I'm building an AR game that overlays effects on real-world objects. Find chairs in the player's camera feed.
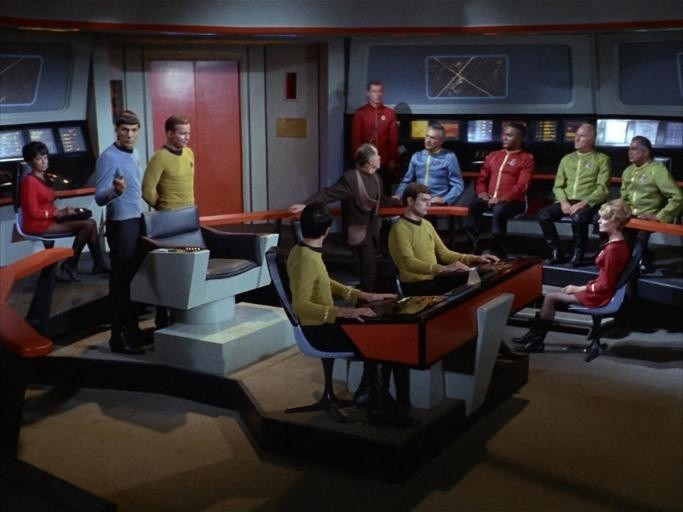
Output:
[264,246,368,426]
[569,243,646,363]
[14,160,67,284]
[373,215,404,287]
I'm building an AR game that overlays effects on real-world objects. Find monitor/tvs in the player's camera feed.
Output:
[410,119,683,148]
[0,129,26,159]
[29,128,58,155]
[58,125,87,153]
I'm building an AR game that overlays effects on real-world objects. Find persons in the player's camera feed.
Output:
[393,122,466,205]
[350,79,398,193]
[143,114,196,325]
[618,135,682,263]
[19,140,105,279]
[93,109,149,355]
[292,143,385,267]
[389,180,501,353]
[512,199,635,353]
[468,115,536,252]
[540,122,614,266]
[286,204,399,417]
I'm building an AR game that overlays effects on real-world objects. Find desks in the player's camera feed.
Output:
[341,256,543,427]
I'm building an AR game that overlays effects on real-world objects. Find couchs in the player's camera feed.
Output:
[112,206,298,378]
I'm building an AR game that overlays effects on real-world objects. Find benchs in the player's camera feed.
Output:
[0,246,79,359]
[599,213,682,237]
[196,205,470,244]
[454,171,683,225]
